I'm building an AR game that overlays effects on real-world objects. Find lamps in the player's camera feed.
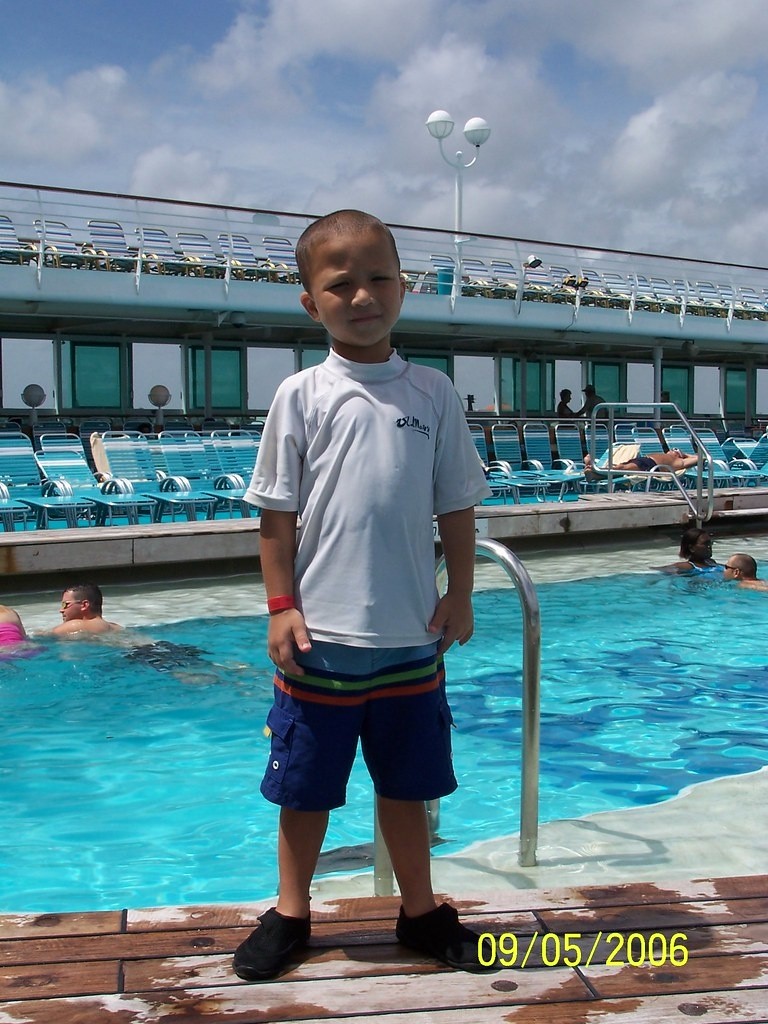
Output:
[517,255,543,315]
[562,274,589,330]
[680,338,699,357]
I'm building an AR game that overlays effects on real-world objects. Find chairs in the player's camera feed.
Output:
[430,254,768,322]
[0,215,303,285]
[0,418,768,533]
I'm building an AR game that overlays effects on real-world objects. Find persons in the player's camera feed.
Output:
[583,448,699,483]
[574,384,608,427]
[0,603,27,645]
[34,583,123,634]
[233,209,491,983]
[556,389,575,428]
[675,529,757,580]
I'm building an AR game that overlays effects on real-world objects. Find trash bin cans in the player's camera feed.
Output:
[437,268,453,296]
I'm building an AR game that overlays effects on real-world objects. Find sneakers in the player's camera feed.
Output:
[231,906,314,979]
[394,901,500,967]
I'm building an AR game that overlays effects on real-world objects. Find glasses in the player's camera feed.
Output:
[671,447,683,458]
[61,599,85,610]
[725,563,737,570]
[697,540,714,548]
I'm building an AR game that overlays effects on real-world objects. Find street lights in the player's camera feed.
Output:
[426,110,490,296]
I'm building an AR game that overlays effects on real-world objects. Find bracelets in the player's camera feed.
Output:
[266,596,295,612]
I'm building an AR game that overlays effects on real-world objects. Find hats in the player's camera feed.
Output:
[582,385,595,391]
